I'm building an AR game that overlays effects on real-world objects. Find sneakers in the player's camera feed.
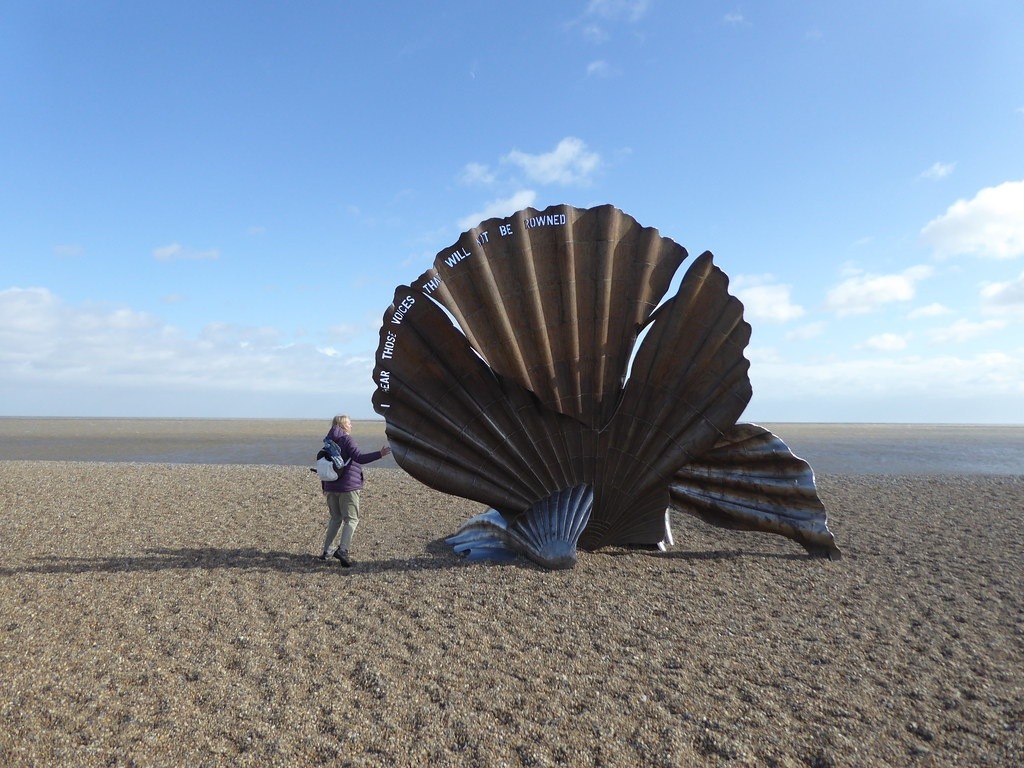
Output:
[318,552,330,563]
[333,545,353,567]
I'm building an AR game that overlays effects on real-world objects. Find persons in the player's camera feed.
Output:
[320,416,392,567]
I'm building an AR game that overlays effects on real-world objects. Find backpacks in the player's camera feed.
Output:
[310,438,352,481]
